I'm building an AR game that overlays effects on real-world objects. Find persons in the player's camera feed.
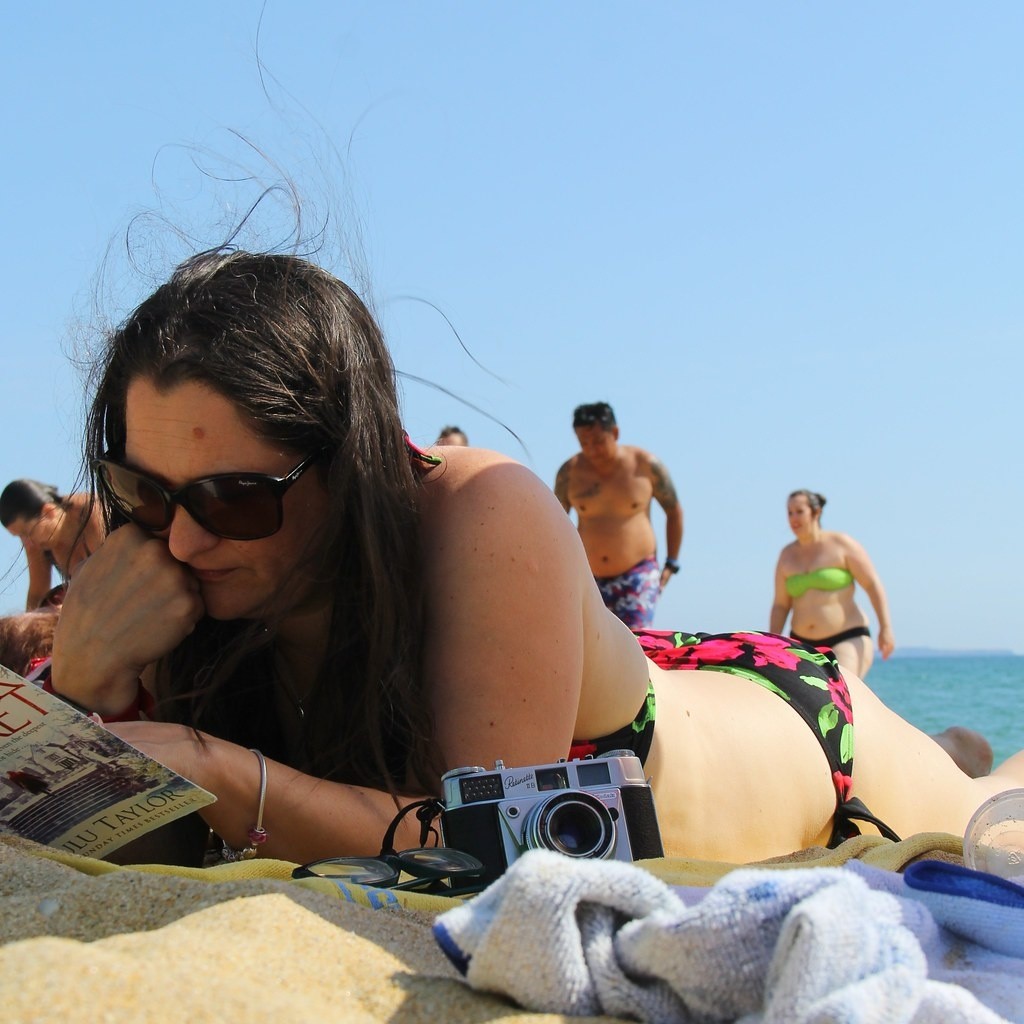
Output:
[47,244,1024,863]
[768,490,894,680]
[554,401,684,631]
[437,426,468,446]
[0,479,112,612]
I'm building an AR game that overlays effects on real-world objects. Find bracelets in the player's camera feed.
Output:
[216,747,268,862]
[40,673,154,721]
[665,558,680,573]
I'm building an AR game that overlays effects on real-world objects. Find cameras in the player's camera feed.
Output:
[437,749,665,899]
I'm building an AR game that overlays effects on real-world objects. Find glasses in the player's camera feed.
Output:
[90,448,326,542]
[291,847,499,897]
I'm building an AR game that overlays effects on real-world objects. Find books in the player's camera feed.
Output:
[0,663,218,861]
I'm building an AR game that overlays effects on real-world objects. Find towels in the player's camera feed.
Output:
[432,847,1024,1024]
